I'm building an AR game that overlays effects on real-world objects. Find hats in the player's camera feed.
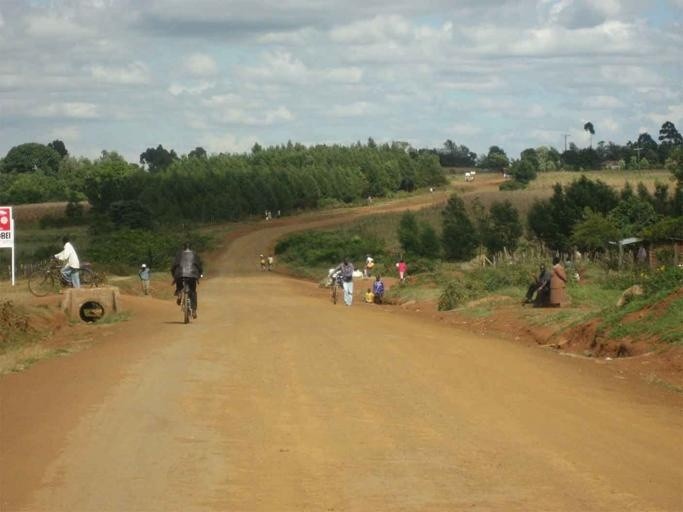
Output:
[142,264,146,268]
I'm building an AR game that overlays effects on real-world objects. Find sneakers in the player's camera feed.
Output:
[176,298,180,305]
[192,311,196,319]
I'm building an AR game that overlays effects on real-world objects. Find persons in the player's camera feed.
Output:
[50,236,81,289]
[170,241,203,319]
[260,253,274,271]
[524,257,581,308]
[362,253,407,305]
[636,243,647,265]
[138,263,152,295]
[329,257,354,305]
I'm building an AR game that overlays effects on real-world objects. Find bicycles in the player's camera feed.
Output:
[178,283,194,325]
[329,276,341,303]
[28,257,95,297]
